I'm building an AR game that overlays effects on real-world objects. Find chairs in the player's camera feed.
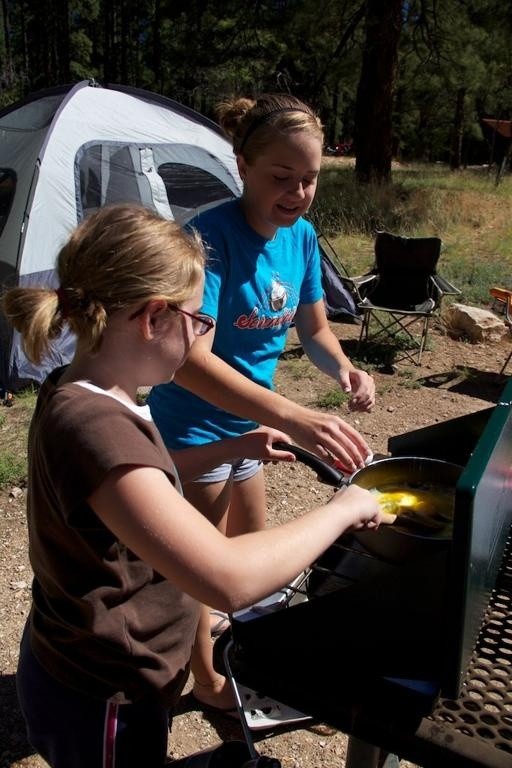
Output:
[353,229,461,366]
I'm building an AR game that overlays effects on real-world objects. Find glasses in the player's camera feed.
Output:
[130,294,213,337]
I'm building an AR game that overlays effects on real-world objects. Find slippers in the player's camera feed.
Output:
[206,609,231,632]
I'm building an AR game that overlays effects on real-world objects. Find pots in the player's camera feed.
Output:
[272,440,469,569]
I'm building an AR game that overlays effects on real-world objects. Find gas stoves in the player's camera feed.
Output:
[211,376,512,701]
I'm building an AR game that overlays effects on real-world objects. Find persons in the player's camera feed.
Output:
[145,95,377,723]
[0,199,384,768]
